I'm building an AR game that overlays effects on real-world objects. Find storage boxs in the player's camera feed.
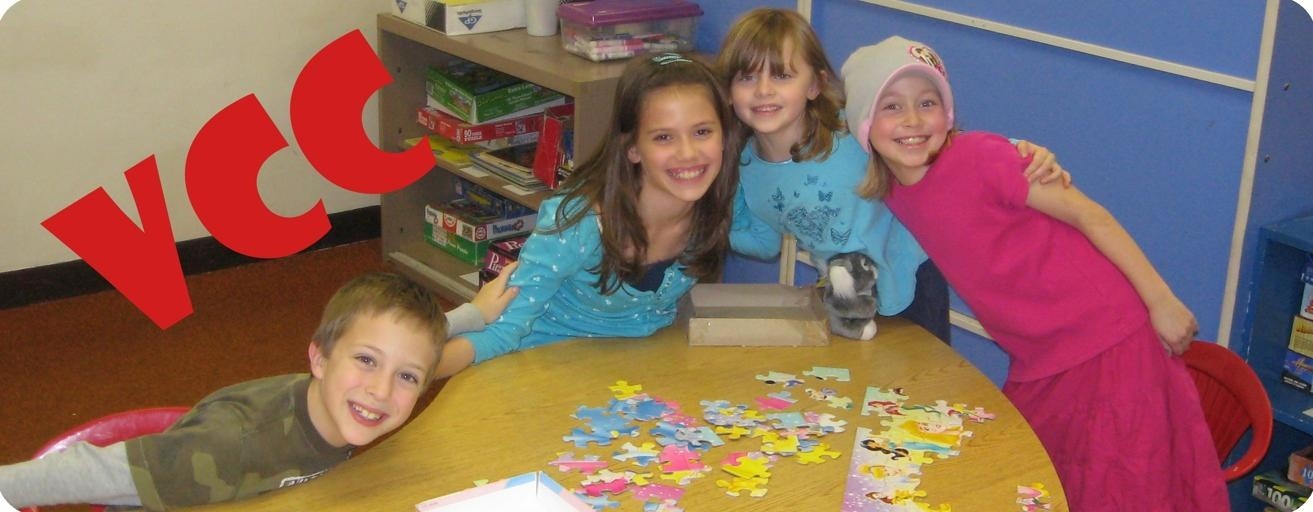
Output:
[560,1,704,61]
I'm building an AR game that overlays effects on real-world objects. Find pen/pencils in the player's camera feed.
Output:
[559,33,694,63]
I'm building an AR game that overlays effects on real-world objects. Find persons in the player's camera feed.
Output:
[839,32,1233,511]
[1,260,520,508]
[431,49,744,383]
[714,6,1072,341]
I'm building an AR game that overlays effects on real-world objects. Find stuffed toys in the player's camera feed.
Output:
[545,366,1056,511]
[821,251,877,341]
[546,365,1055,511]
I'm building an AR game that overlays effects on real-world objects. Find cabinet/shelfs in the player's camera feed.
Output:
[373,9,722,314]
[1249,212,1313,512]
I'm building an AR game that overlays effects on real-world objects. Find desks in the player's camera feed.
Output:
[210,306,1072,512]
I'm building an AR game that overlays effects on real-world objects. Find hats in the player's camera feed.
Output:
[841,36,955,154]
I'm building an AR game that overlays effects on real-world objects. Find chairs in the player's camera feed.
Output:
[1171,336,1278,486]
[2,405,204,509]
